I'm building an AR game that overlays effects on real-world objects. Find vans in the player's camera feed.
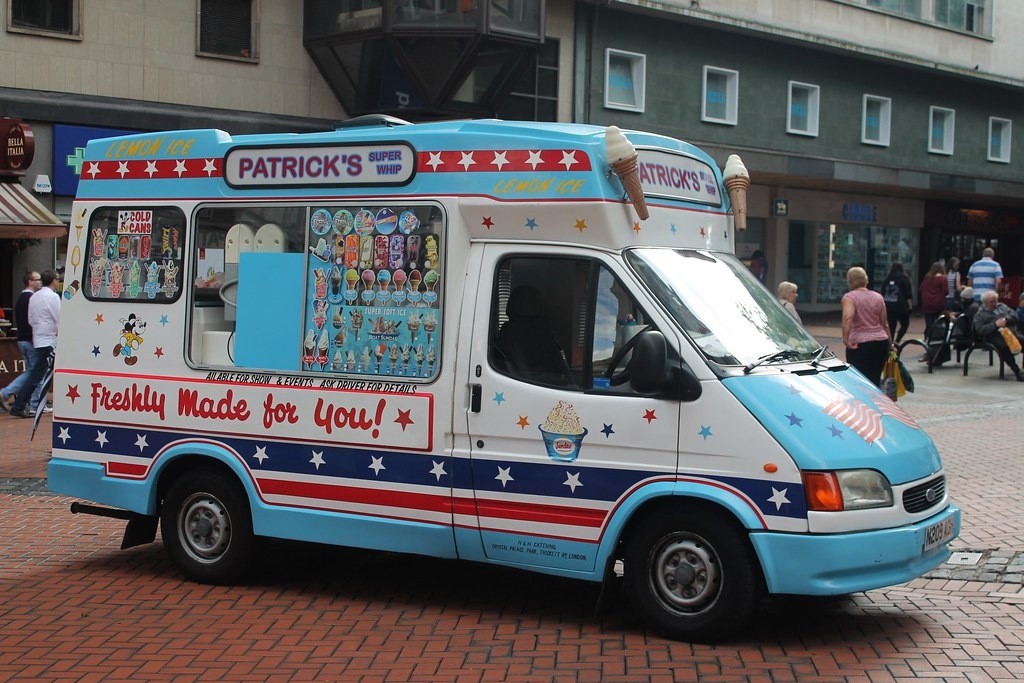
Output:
[46,103,959,629]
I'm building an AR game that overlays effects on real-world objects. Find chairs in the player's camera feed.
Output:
[497,285,584,401]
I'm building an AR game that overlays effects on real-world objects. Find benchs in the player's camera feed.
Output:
[963,313,1024,379]
[927,314,964,375]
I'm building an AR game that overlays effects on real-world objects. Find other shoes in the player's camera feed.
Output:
[1016,370,1024,381]
[919,353,942,366]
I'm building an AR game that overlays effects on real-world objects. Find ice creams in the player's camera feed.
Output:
[91,227,107,257]
[722,153,751,231]
[64,207,88,301]
[89,257,180,297]
[304,208,437,377]
[539,400,589,464]
[604,124,650,223]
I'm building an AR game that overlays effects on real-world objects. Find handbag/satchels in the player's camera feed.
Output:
[880,351,914,402]
[999,327,1022,355]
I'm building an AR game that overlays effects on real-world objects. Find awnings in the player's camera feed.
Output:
[1,183,68,239]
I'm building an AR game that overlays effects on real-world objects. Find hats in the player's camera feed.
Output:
[960,287,974,299]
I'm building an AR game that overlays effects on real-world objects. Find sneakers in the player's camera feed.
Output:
[0,391,10,411]
[30,405,53,414]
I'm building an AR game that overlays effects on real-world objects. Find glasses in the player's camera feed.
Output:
[55,277,60,280]
[32,278,42,281]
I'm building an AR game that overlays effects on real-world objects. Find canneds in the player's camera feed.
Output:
[107,235,152,260]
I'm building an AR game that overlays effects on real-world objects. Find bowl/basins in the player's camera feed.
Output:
[538,423,588,463]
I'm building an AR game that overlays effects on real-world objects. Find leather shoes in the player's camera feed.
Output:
[11,407,36,417]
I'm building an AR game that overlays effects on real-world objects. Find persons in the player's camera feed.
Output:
[0,271,54,416]
[841,267,897,392]
[776,281,802,328]
[880,262,913,349]
[920,262,949,336]
[918,287,980,368]
[973,290,1024,382]
[751,250,768,287]
[946,247,1024,337]
[9,270,62,418]
